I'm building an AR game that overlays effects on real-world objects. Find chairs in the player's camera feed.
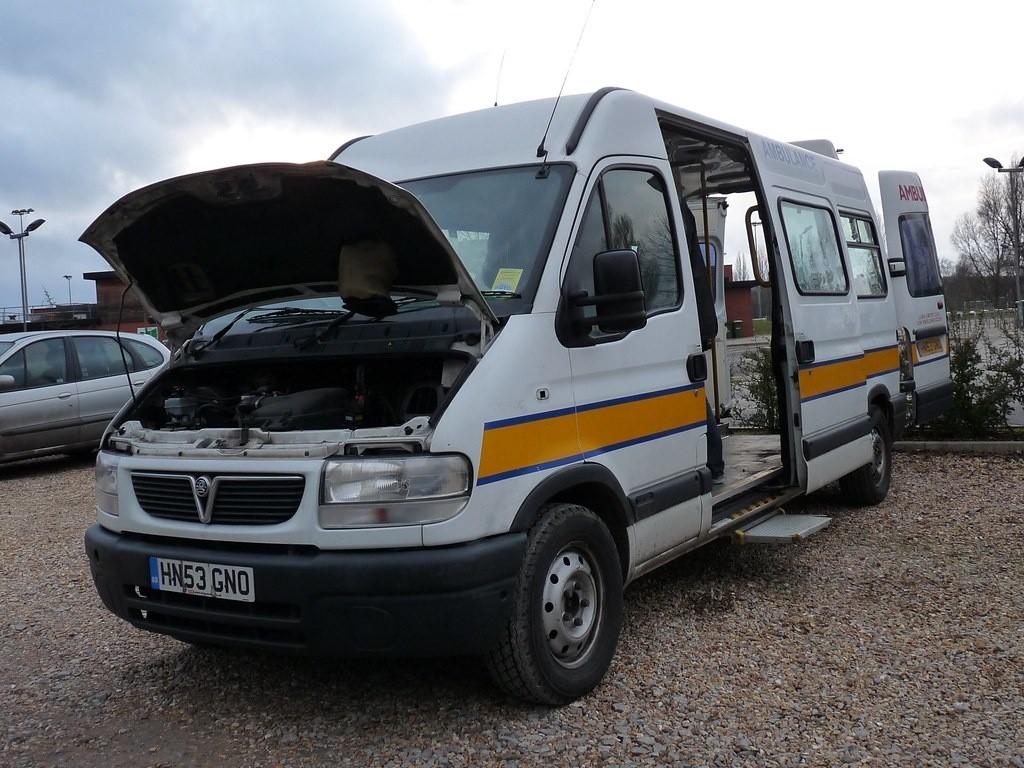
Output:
[3,345,66,388]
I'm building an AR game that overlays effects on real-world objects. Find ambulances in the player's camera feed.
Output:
[84,80,959,714]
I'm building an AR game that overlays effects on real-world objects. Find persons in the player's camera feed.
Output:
[682,202,726,485]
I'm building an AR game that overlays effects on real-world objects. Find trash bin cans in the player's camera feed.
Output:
[732,320,744,338]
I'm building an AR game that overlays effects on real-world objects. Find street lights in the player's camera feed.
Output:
[982,154,1024,327]
[61,272,73,305]
[0,205,47,322]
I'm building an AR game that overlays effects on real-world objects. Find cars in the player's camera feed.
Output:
[0,328,173,470]
